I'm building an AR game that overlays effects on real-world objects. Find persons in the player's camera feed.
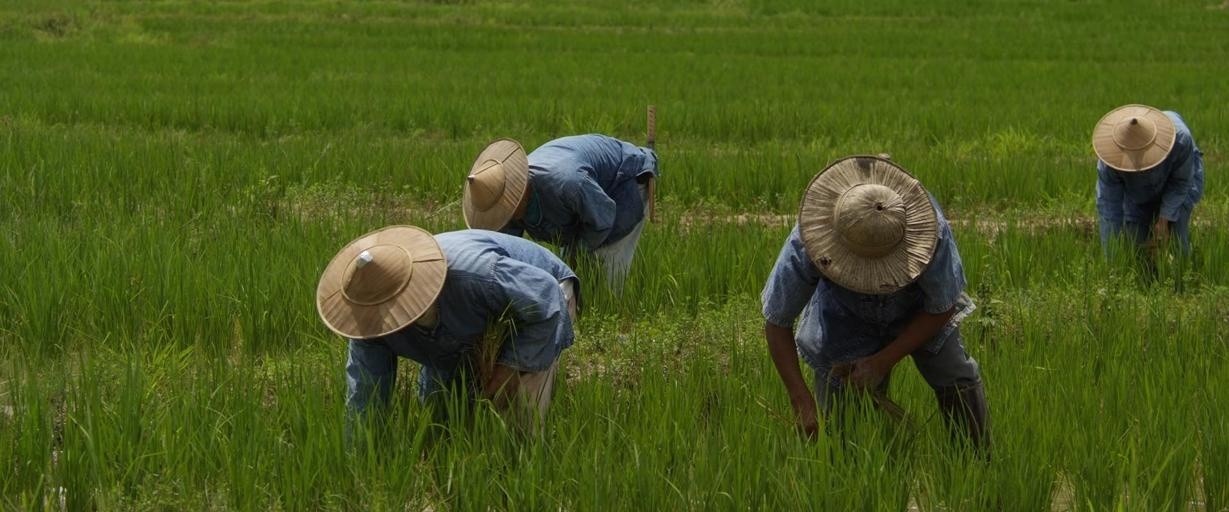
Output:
[1092,104,1204,295]
[462,134,660,298]
[760,155,997,468]
[316,224,583,464]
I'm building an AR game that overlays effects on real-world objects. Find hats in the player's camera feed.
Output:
[460,136,531,235]
[798,152,942,297]
[1090,102,1178,173]
[313,224,449,341]
